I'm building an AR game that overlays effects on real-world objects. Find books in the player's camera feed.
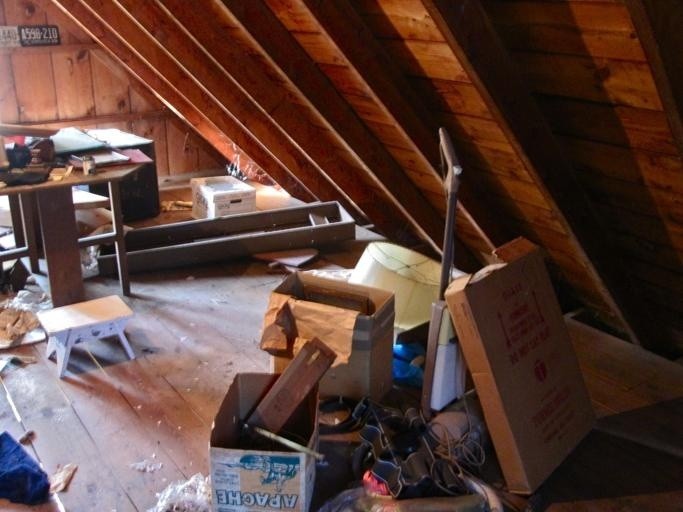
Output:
[50,116,156,171]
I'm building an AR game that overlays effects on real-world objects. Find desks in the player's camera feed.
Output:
[0,162,143,311]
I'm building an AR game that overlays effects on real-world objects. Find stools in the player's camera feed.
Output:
[33,292,138,378]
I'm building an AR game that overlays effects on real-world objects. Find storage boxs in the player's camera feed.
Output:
[206,371,320,511]
[188,174,256,221]
[260,271,396,405]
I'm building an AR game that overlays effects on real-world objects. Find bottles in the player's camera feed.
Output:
[82,155,96,175]
